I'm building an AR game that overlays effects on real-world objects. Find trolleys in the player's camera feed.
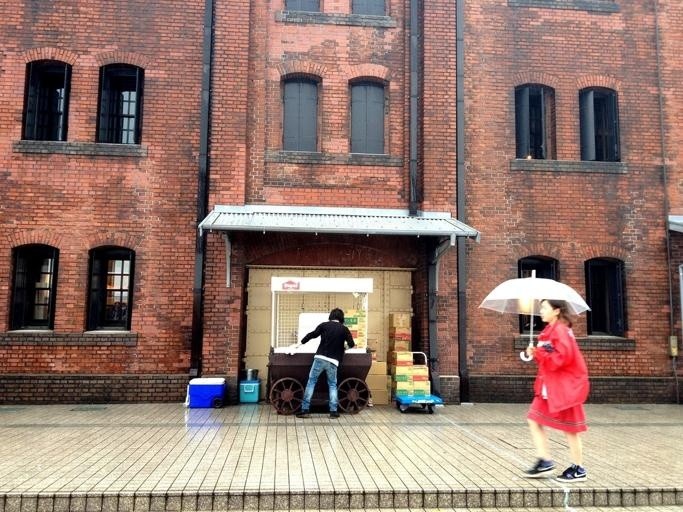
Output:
[265,346,376,415]
[391,350,443,413]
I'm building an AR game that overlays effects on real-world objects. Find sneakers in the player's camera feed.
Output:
[522,460,556,478]
[330,412,339,419]
[296,411,308,417]
[556,465,586,482]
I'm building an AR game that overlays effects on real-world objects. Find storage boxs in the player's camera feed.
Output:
[391,364,413,381]
[387,352,413,366]
[389,328,411,340]
[369,389,391,404]
[240,379,261,403]
[365,374,391,389]
[413,365,428,381]
[368,362,386,374]
[392,381,414,396]
[389,340,409,352]
[413,381,430,396]
[189,378,228,407]
[389,313,409,328]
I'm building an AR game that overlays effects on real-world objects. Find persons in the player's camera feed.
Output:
[521,299,590,482]
[285,307,355,419]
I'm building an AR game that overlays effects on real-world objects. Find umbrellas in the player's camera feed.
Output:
[476,269,592,362]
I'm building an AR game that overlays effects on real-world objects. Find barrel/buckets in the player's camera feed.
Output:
[242,369,260,381]
